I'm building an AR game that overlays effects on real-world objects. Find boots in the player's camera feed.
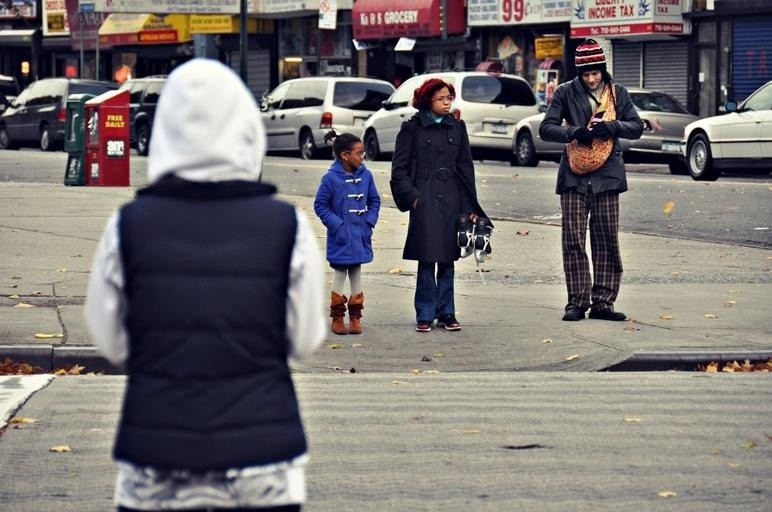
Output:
[348,292,365,336]
[329,291,348,336]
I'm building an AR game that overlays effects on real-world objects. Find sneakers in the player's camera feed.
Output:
[475,217,495,254]
[455,213,472,249]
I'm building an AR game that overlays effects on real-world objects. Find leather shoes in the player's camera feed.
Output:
[561,306,585,321]
[436,316,463,331]
[415,321,436,331]
[588,305,628,322]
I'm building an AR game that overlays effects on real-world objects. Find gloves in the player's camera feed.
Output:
[590,120,619,141]
[570,128,595,150]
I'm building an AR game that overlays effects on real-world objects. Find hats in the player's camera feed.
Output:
[574,39,607,71]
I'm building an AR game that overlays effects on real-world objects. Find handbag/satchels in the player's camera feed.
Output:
[389,121,418,214]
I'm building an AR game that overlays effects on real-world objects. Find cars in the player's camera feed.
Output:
[679,79,772,181]
[511,86,703,175]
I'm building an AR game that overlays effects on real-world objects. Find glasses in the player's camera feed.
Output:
[342,149,365,160]
[431,96,455,105]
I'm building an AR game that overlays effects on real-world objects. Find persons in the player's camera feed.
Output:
[538,39,646,322]
[389,79,481,333]
[314,127,380,334]
[81,57,330,512]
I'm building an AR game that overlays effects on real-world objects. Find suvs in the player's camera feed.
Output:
[117,74,169,156]
[259,75,396,160]
[360,69,541,162]
[0,76,120,152]
